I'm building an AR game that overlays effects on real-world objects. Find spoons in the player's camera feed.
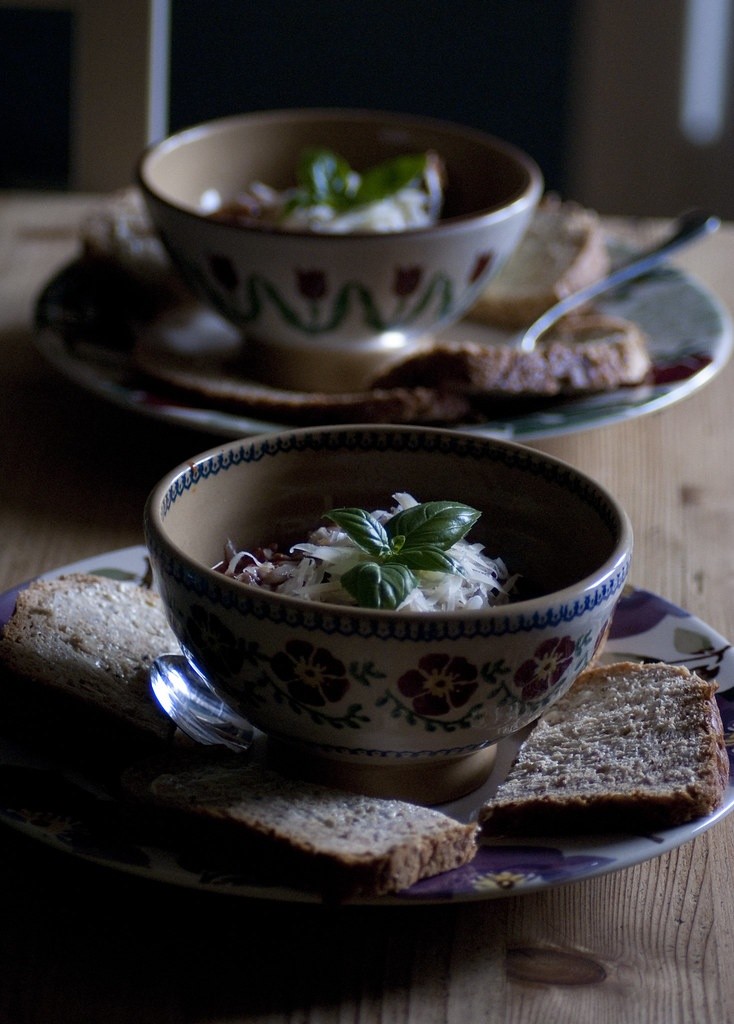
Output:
[148,653,275,751]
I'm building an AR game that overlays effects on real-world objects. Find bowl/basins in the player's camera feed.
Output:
[136,113,539,389]
[143,425,633,807]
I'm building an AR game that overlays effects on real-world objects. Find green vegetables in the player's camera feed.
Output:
[321,500,484,612]
[263,142,426,217]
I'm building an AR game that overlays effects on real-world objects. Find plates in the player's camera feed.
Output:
[0,544,734,905]
[32,236,734,440]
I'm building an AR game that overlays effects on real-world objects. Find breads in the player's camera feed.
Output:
[77,184,649,425]
[0,574,732,895]
